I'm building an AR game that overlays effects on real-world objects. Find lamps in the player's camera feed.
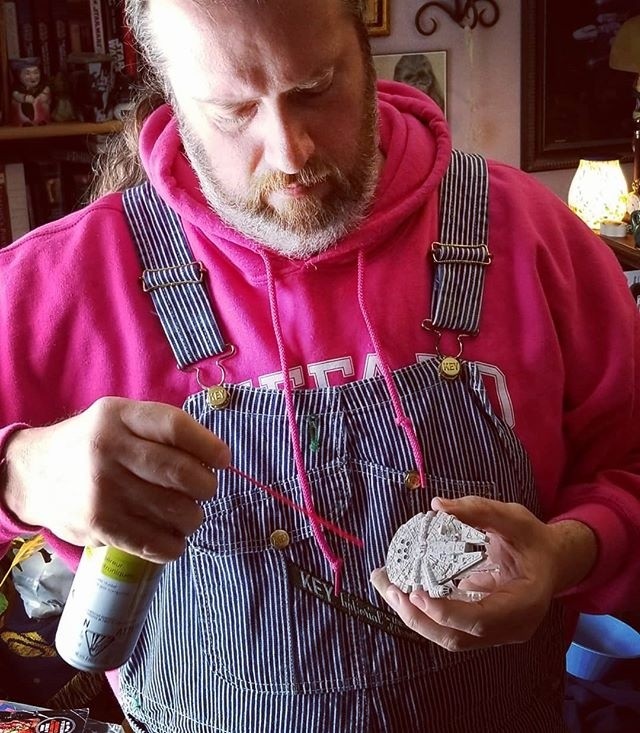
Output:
[567,152,629,229]
[609,16,640,214]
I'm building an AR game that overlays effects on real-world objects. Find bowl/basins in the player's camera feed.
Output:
[566,613,640,681]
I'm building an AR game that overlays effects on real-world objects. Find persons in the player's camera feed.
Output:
[0,0,640,732]
[11,56,57,126]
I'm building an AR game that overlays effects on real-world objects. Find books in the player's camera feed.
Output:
[0,161,97,249]
[0,0,155,122]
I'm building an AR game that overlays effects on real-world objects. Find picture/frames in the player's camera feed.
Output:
[521,0,640,173]
[371,50,448,121]
